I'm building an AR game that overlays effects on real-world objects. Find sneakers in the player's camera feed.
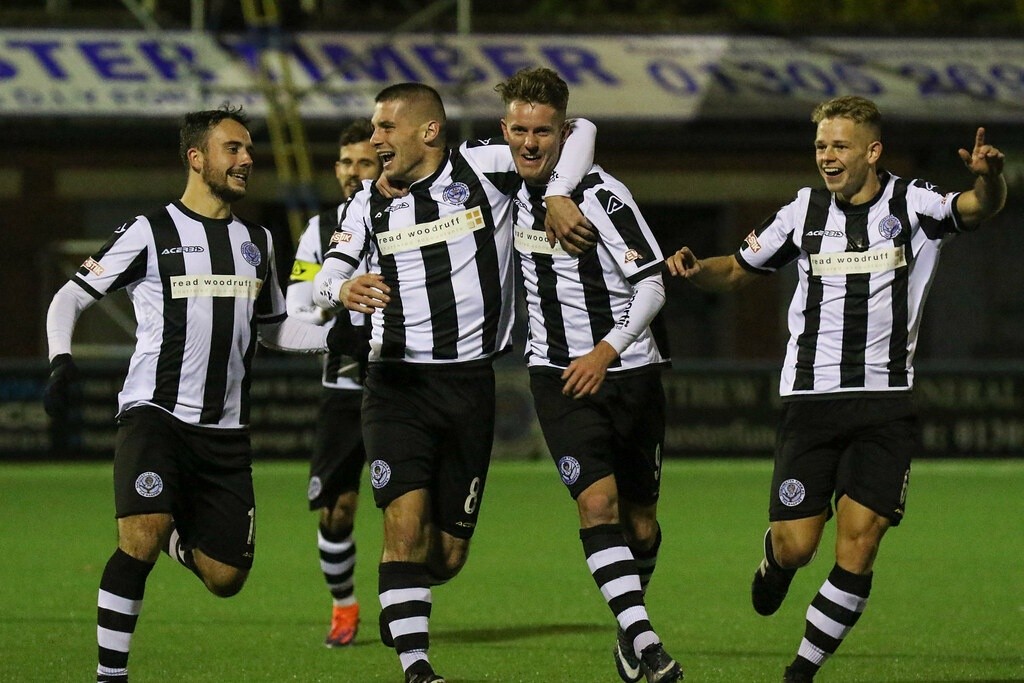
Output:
[406,672,447,683]
[613,620,645,683]
[640,642,684,683]
[379,610,399,647]
[325,603,361,648]
[752,526,817,615]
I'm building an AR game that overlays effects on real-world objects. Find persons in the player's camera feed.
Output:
[289,68,685,683]
[42,103,371,683]
[665,96,1007,683]
